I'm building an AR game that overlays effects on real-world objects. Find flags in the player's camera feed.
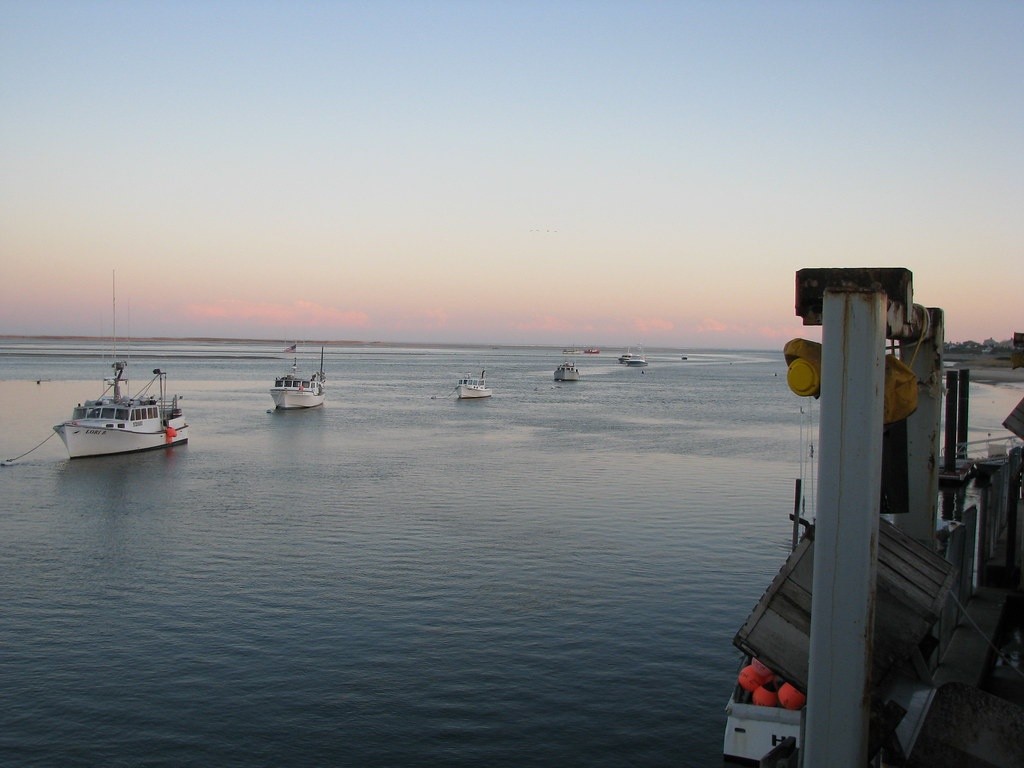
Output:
[283,344,296,353]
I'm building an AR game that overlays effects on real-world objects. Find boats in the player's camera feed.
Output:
[562,343,581,354]
[583,348,600,354]
[52,270,190,459]
[618,343,649,366]
[681,352,688,360]
[554,359,580,381]
[724,265,1024,768]
[270,337,326,409]
[455,359,492,398]
